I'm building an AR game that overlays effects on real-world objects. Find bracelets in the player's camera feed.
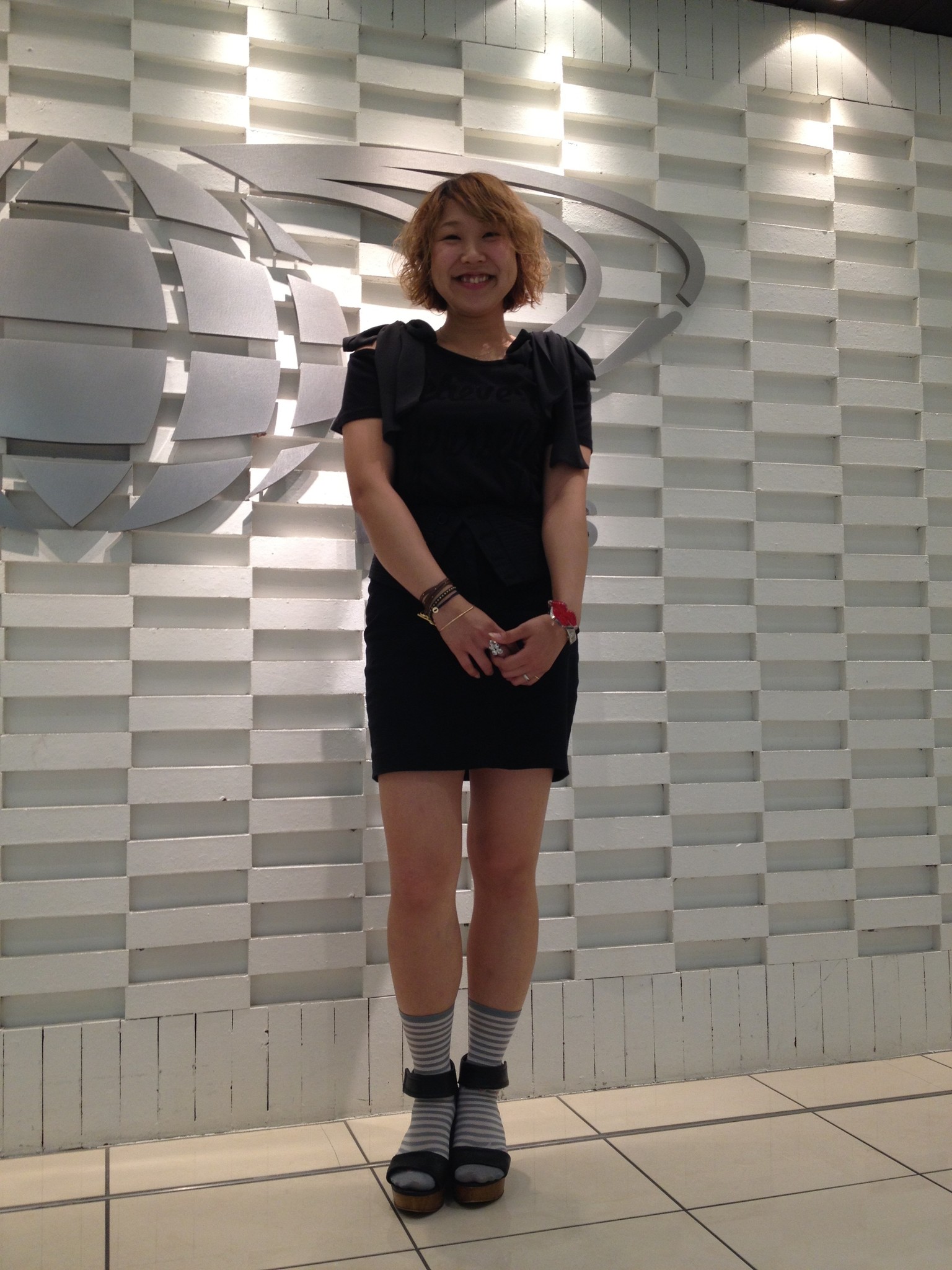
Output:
[548,600,579,644]
[418,577,459,625]
[439,606,474,632]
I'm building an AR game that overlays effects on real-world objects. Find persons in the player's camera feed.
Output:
[330,171,597,1219]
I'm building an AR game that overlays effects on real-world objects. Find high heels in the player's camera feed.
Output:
[386,1059,453,1214]
[450,1062,509,1203]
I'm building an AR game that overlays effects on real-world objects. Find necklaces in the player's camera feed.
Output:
[439,328,509,360]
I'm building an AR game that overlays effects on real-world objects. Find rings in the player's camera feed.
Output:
[535,676,540,680]
[523,674,530,680]
[489,640,503,655]
